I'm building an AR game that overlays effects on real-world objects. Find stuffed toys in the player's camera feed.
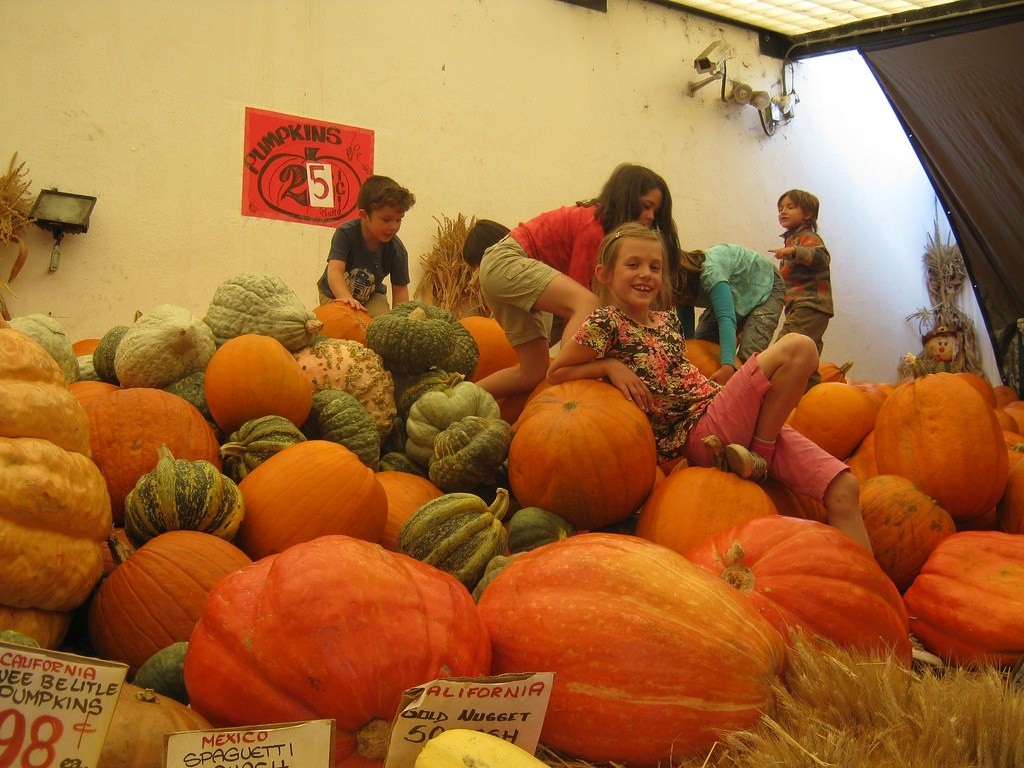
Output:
[921,326,960,375]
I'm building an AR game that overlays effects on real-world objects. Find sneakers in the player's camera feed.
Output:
[724,442,767,482]
[908,630,944,670]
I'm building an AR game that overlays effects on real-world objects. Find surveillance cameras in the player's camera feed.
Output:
[694,39,735,74]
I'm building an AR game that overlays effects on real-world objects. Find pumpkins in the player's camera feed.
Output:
[1,274,1024,765]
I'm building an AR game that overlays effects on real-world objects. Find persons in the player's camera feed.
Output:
[463,217,515,317]
[768,188,835,398]
[473,162,682,405]
[318,174,417,321]
[546,220,874,559]
[672,242,786,388]
